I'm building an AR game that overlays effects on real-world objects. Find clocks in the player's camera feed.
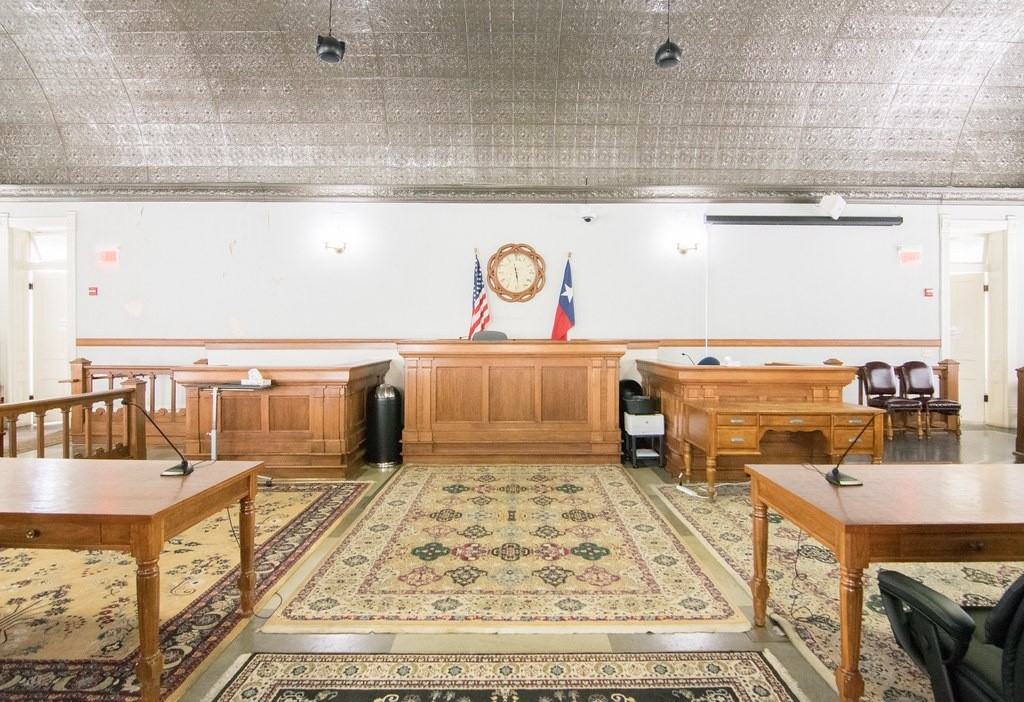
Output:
[487,243,546,303]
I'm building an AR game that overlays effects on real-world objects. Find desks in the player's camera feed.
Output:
[744,463,1024,702]
[0,458,265,702]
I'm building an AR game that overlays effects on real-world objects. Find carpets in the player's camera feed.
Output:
[202,647,809,702]
[0,479,377,702]
[651,481,1024,702]
[256,463,753,635]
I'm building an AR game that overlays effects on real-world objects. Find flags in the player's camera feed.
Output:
[469,258,490,340]
[551,259,575,341]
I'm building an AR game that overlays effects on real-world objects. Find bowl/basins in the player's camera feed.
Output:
[624,399,656,414]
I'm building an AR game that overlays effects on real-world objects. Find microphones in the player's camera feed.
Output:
[826,408,894,486]
[121,399,194,476]
[682,353,695,365]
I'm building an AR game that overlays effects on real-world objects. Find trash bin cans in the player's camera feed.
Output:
[619,379,644,460]
[364,384,401,468]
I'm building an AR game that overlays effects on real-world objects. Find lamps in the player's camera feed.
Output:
[315,0,345,63]
[655,0,682,70]
[819,194,846,220]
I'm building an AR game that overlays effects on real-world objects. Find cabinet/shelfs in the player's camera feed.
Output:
[624,412,664,468]
[681,398,886,504]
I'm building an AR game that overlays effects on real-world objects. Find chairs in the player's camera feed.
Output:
[878,569,1024,702]
[856,361,962,442]
